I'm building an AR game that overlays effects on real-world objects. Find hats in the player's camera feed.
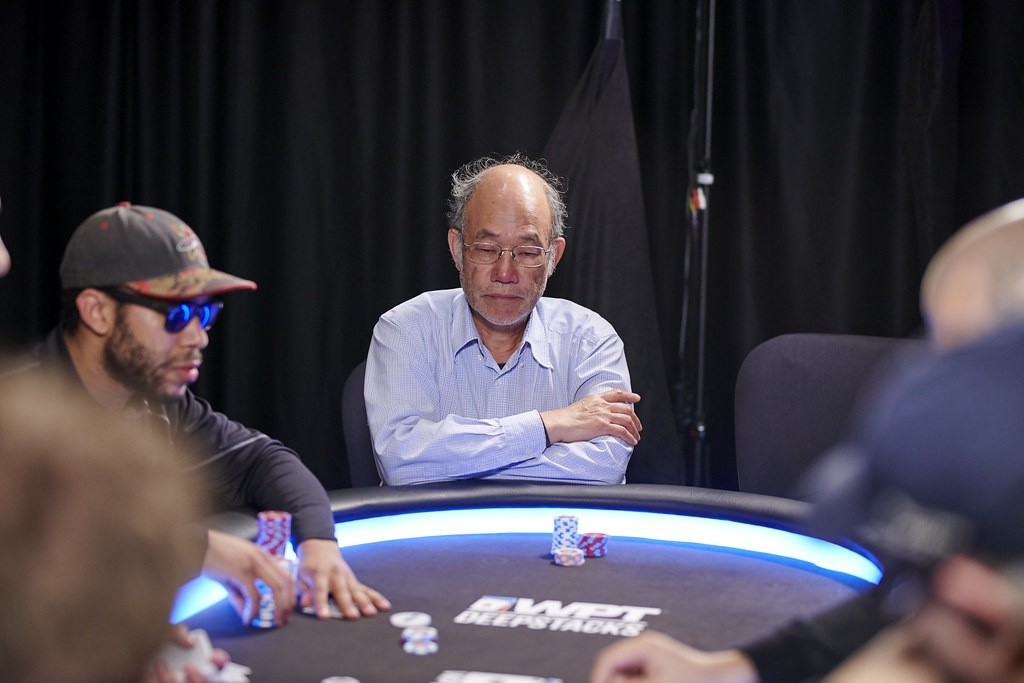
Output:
[59,201,258,298]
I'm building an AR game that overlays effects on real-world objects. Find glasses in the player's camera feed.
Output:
[461,234,556,268]
[106,290,225,333]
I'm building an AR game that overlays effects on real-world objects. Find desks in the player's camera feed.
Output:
[167,477,880,683]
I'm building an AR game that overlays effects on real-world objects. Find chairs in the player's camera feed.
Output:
[734,333,922,506]
[340,364,383,486]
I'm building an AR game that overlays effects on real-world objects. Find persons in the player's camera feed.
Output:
[0,202,390,626]
[0,371,230,683]
[361,157,642,487]
[593,196,1024,683]
